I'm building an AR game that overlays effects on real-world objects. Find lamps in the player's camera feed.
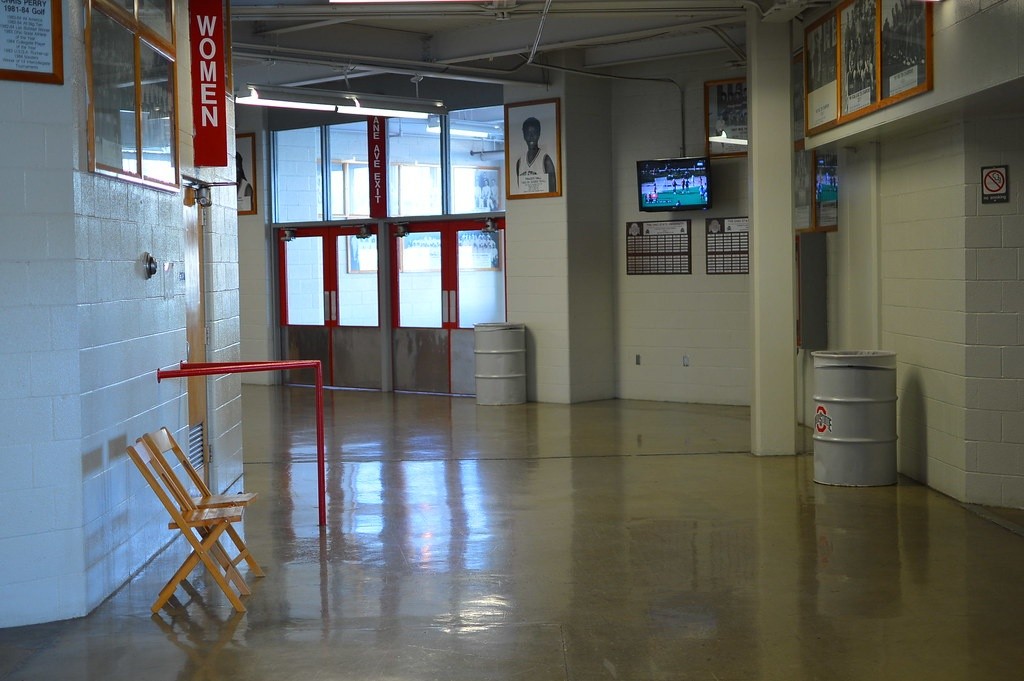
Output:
[235,82,450,120]
[425,116,502,139]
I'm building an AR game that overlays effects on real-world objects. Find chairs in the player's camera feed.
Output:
[123,436,252,614]
[141,426,267,591]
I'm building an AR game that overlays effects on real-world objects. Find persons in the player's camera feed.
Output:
[794,150,810,204]
[671,176,707,205]
[807,32,824,94]
[844,0,876,91]
[642,163,683,175]
[714,88,747,148]
[882,0,925,66]
[357,232,498,267]
[816,170,838,200]
[236,151,253,211]
[645,183,658,204]
[474,168,499,211]
[516,117,556,193]
[91,6,172,148]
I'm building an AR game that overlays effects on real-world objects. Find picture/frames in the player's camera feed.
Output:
[703,77,749,160]
[803,0,934,138]
[503,97,562,200]
[236,132,257,216]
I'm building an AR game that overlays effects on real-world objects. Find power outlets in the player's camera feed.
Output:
[683,354,690,367]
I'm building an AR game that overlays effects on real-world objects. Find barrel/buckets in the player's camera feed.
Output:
[472,322,526,405]
[810,350,899,487]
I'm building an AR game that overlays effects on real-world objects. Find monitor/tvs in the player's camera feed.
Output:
[635,156,712,211]
[813,152,839,205]
